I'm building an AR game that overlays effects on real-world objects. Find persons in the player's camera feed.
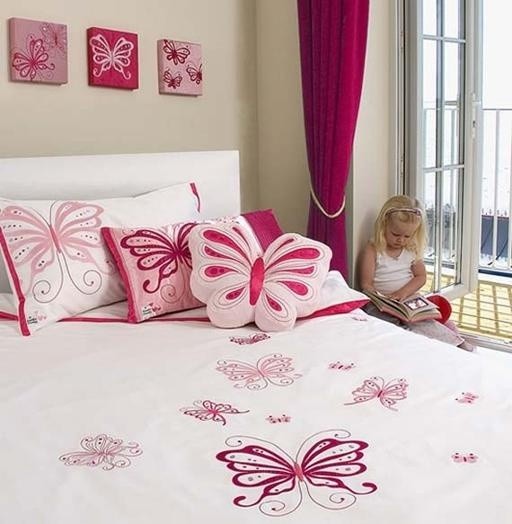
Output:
[357,194,472,351]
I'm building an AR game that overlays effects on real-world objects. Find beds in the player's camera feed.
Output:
[2,148,511,524]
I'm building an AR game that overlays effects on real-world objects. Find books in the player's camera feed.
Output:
[363,288,444,323]
